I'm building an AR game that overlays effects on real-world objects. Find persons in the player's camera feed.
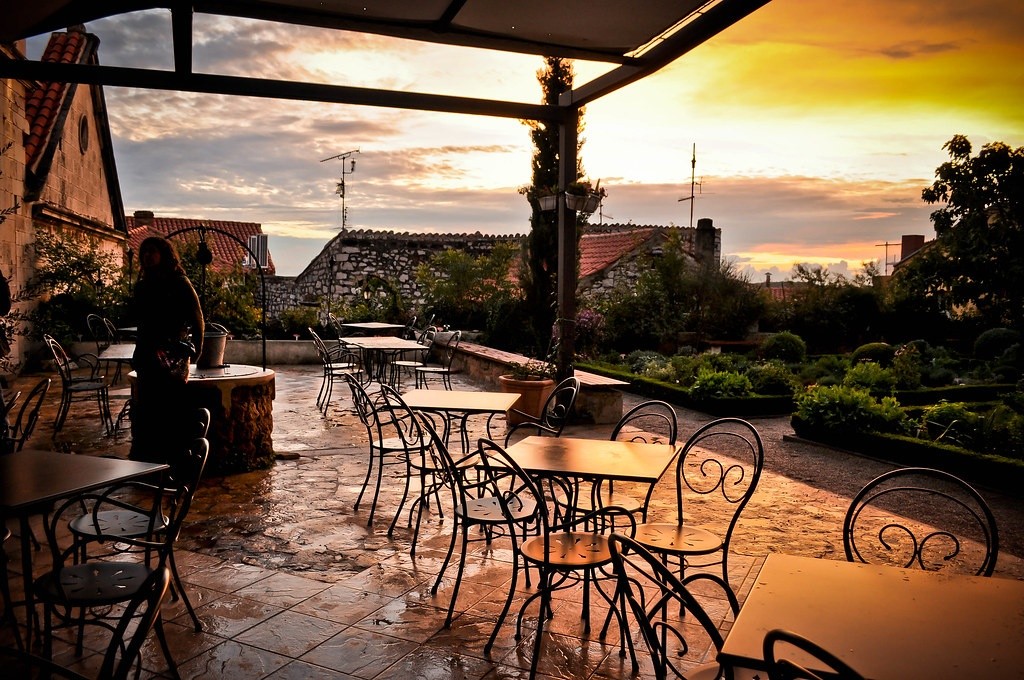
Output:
[0,268,11,438]
[130,236,205,488]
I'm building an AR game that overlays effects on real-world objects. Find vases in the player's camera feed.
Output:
[438,330,456,341]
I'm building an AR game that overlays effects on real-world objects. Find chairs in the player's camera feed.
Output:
[305,322,999,680]
[0,313,217,680]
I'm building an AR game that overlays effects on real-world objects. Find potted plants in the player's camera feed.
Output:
[496,356,558,426]
[518,181,558,211]
[566,181,607,213]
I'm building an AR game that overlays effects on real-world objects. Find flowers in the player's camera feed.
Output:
[442,323,451,332]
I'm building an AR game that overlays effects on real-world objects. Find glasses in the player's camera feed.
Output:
[142,247,160,254]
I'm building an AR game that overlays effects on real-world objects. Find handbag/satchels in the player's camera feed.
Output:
[146,340,190,381]
[0,317,10,357]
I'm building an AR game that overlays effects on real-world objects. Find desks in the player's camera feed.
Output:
[127,365,276,474]
[718,551,1024,680]
[342,323,406,370]
[0,449,184,680]
[97,343,136,374]
[380,388,521,505]
[338,337,430,381]
[474,433,682,620]
